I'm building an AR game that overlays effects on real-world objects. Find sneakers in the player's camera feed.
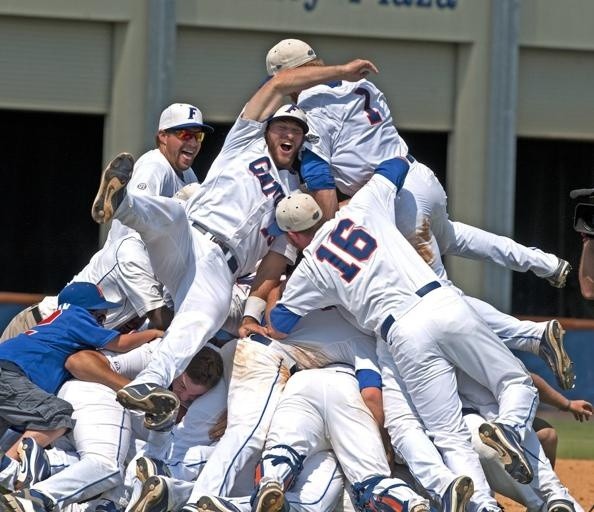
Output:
[141,406,178,429]
[115,382,178,410]
[546,257,570,286]
[91,153,134,226]
[539,320,577,393]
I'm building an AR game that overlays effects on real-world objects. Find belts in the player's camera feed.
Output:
[404,152,415,167]
[30,302,42,324]
[191,222,242,279]
[379,281,441,342]
[247,333,295,374]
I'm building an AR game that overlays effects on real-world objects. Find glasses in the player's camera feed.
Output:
[166,128,204,143]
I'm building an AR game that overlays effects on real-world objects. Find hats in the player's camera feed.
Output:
[265,38,315,78]
[266,104,309,136]
[57,282,121,309]
[275,193,322,233]
[156,103,214,136]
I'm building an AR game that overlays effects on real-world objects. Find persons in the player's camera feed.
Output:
[104,100,216,245]
[91,58,382,418]
[265,38,577,393]
[1,155,594,512]
